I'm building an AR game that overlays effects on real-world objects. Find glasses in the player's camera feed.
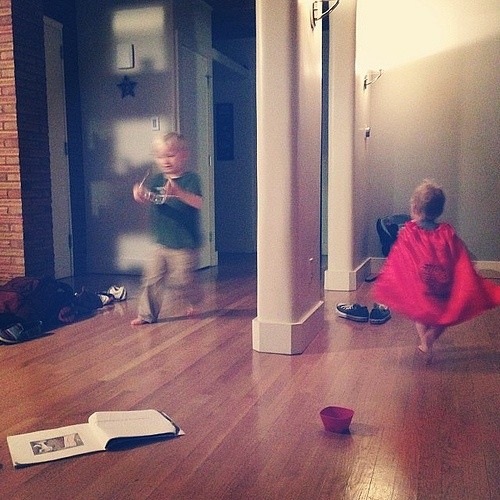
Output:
[136,172,170,205]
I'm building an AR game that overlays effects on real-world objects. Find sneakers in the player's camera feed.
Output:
[336,301,370,322]
[368,303,392,324]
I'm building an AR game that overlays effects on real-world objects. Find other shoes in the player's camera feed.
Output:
[98,284,127,304]
[0,319,44,345]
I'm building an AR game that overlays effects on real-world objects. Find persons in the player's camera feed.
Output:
[131,132,205,325]
[369,178,500,362]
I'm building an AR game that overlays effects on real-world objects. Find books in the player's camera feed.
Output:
[7,409,186,469]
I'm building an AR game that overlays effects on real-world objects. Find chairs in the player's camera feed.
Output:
[376,213,412,257]
